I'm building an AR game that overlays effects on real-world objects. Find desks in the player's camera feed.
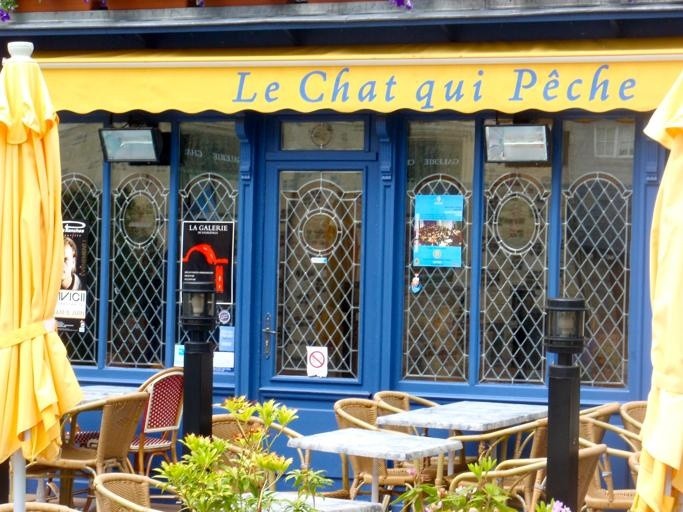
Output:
[214,488,381,512]
[287,427,462,512]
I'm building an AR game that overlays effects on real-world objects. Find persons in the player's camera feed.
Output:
[62,237,82,289]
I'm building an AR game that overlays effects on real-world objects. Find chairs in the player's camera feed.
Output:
[212,412,310,492]
[0,366,195,512]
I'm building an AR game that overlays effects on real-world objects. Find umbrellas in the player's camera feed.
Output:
[0,42,84,511]
[631,67,683,512]
